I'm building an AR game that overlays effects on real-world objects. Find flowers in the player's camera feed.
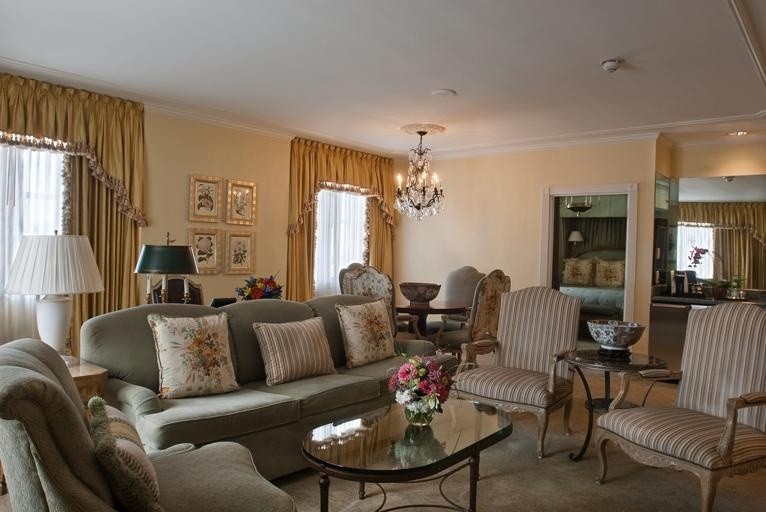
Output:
[389,425,450,469]
[387,352,458,422]
[236,272,285,300]
[688,246,721,268]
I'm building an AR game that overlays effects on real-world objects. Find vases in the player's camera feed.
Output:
[405,408,432,426]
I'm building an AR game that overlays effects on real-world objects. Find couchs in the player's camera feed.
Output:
[80,296,458,481]
[559,250,625,311]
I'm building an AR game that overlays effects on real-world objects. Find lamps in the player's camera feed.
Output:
[5,230,200,368]
[563,196,601,218]
[392,123,446,222]
[568,231,584,257]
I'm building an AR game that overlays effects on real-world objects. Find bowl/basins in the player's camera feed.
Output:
[405,305,434,310]
[400,283,442,304]
[598,356,632,365]
[586,318,646,356]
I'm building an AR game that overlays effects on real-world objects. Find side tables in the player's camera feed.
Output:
[68,359,108,405]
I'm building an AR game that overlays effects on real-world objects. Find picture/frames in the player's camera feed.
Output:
[188,173,257,275]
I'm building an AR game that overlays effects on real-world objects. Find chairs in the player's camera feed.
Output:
[338,263,512,369]
[0,338,298,512]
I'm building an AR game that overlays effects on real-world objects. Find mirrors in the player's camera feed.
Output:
[539,182,639,364]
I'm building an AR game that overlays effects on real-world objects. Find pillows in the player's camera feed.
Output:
[87,396,166,512]
[562,257,624,287]
